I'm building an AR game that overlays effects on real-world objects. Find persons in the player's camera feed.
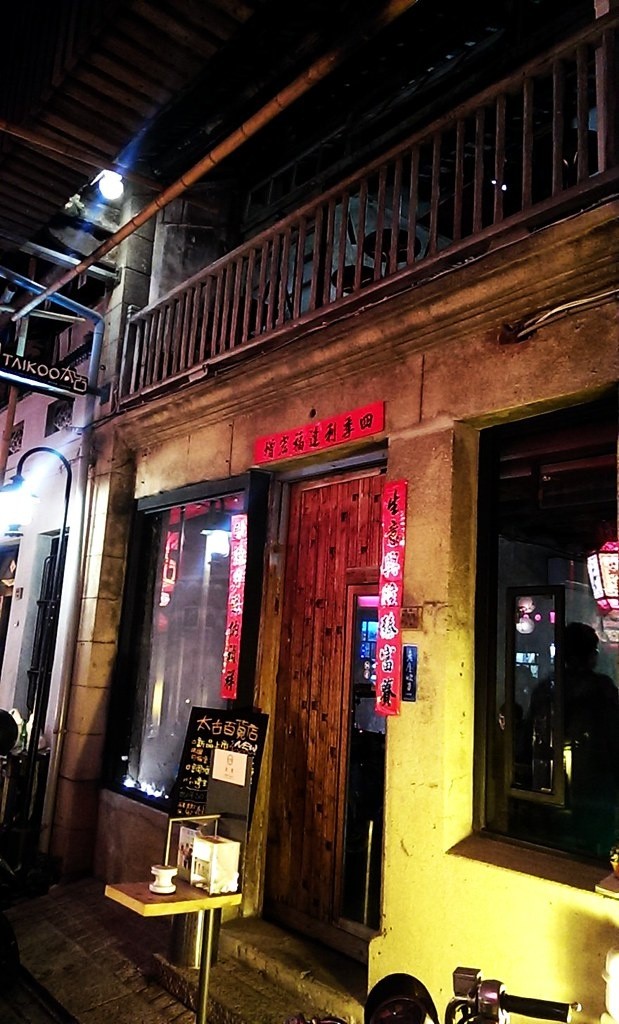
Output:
[520,622,619,823]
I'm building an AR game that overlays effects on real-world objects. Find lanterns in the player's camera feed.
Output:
[587,609,619,650]
[586,540,619,619]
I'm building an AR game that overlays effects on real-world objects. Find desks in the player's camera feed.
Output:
[105,878,242,1024]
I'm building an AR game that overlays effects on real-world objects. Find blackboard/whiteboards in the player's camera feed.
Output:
[168,705,270,841]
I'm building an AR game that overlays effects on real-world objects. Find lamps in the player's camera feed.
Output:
[586,541,619,617]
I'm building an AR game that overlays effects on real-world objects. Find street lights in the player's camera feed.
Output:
[1,447,74,885]
[130,501,233,793]
